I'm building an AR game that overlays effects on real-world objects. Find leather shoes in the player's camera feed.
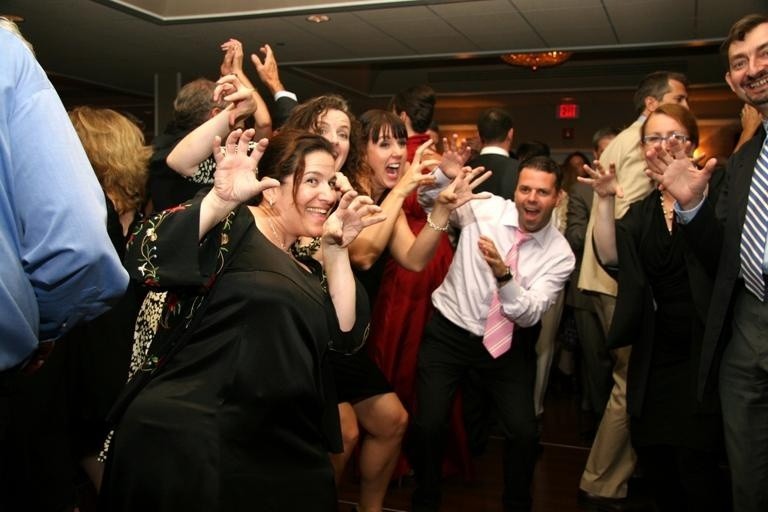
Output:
[411,489,439,512]
[572,492,626,512]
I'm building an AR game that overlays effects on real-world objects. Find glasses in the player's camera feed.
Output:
[644,133,692,147]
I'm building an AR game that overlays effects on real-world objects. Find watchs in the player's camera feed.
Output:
[496,265,516,284]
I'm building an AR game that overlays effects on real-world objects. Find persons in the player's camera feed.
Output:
[411,134,575,512]
[95,75,353,489]
[512,139,590,184]
[64,104,152,256]
[645,14,764,510]
[426,121,444,155]
[576,104,724,509]
[566,128,621,440]
[0,16,129,512]
[149,43,298,209]
[463,110,521,202]
[101,128,386,512]
[220,36,270,143]
[578,72,691,507]
[330,109,492,512]
[369,84,466,481]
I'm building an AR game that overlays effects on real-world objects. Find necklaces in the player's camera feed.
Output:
[259,204,294,256]
[658,191,676,222]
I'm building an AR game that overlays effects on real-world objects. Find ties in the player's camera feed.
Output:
[740,131,768,299]
[482,228,534,359]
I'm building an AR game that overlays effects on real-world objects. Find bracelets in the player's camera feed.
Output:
[426,210,452,231]
[248,87,258,93]
